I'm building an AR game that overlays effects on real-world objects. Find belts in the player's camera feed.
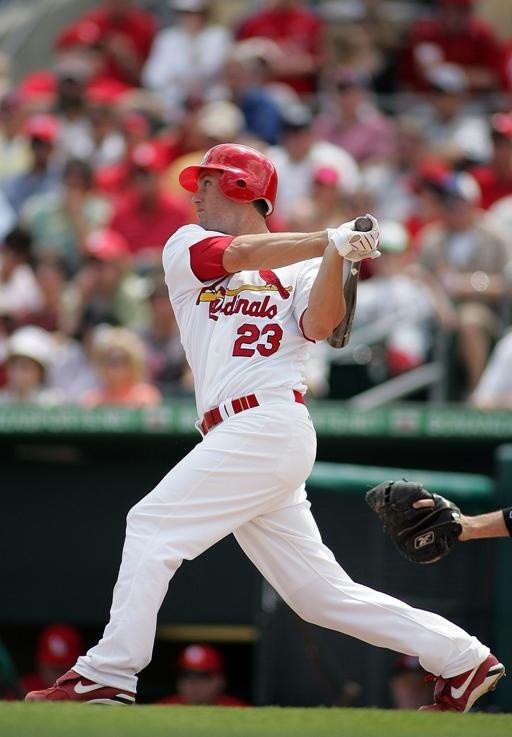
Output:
[200,389,306,436]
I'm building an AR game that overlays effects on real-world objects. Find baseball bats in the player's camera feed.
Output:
[329,218,372,346]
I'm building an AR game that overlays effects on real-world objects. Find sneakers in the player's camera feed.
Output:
[417,653,505,713]
[25,669,137,706]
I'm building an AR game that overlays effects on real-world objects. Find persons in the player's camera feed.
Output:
[387,654,435,705]
[8,623,84,701]
[149,645,255,707]
[24,139,502,714]
[2,1,512,413]
[362,476,511,567]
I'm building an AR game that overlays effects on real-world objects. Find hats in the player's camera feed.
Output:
[40,623,82,664]
[179,646,220,673]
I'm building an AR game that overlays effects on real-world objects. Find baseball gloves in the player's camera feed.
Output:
[366,478,464,566]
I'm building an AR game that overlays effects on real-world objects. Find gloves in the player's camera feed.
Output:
[323,213,381,262]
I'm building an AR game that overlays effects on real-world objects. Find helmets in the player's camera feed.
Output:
[180,143,278,215]
